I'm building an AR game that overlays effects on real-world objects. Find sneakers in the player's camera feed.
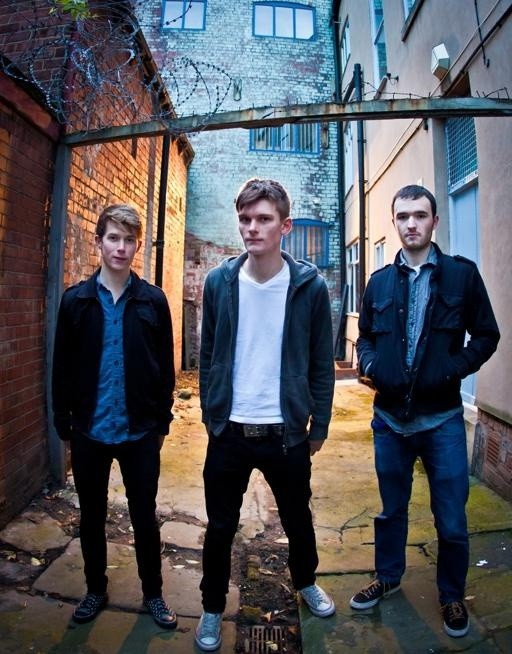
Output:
[143,597,177,629]
[441,601,469,637]
[194,610,222,651]
[299,584,335,617]
[350,579,400,609]
[72,592,108,624]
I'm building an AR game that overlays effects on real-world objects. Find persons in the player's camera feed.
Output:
[192,176,336,652]
[51,202,178,633]
[347,180,501,639]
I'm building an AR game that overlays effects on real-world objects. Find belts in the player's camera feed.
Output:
[228,422,286,438]
